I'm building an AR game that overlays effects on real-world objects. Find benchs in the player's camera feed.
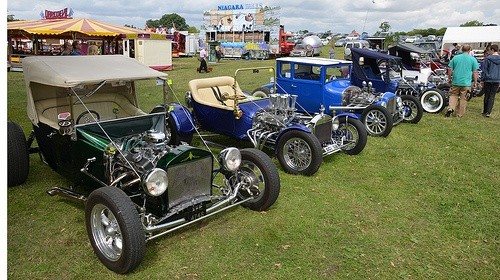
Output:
[35,94,150,130]
[397,55,419,71]
[295,72,314,80]
[362,65,377,79]
[189,76,262,111]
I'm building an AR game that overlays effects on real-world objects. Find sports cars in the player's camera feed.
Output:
[348,47,451,113]
[271,55,425,138]
[151,65,368,178]
[393,38,485,102]
[8,52,281,275]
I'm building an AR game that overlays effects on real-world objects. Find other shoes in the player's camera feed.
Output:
[456,114,463,119]
[445,108,454,118]
[481,112,490,118]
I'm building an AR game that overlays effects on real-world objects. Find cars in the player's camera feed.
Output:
[292,32,445,49]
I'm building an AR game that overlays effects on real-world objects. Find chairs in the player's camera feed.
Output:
[223,48,242,60]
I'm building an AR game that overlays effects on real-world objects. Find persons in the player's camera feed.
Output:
[70,41,83,55]
[61,42,73,55]
[449,43,500,60]
[444,45,480,118]
[199,47,211,73]
[329,45,335,59]
[88,45,99,55]
[480,44,500,117]
[376,45,381,53]
